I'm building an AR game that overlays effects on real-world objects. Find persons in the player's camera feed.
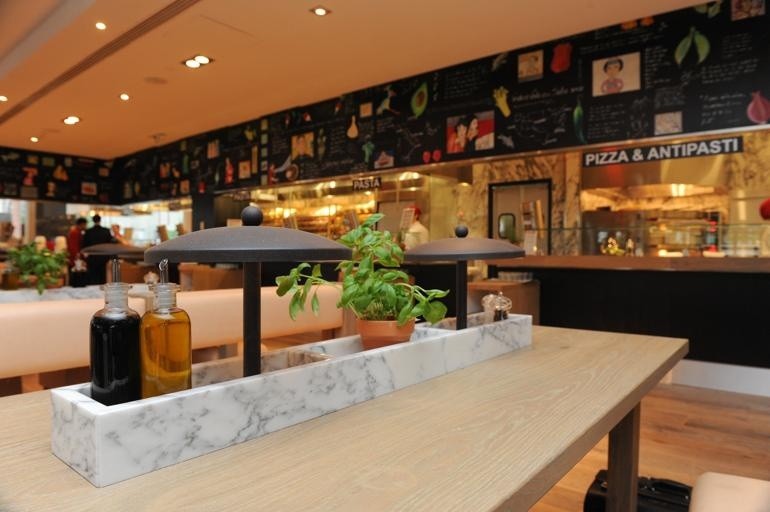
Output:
[111,225,129,244]
[82,214,122,285]
[758,198,769,259]
[404,203,430,250]
[66,216,88,273]
[445,118,468,154]
[465,114,495,153]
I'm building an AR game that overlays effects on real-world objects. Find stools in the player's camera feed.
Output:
[688,471,770,512]
[464,277,539,330]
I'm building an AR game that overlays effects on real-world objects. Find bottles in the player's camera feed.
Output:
[87,257,193,406]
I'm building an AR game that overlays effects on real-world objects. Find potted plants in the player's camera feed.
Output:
[274,210,451,351]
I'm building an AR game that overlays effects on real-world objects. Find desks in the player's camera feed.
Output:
[4,282,349,396]
[2,320,688,511]
